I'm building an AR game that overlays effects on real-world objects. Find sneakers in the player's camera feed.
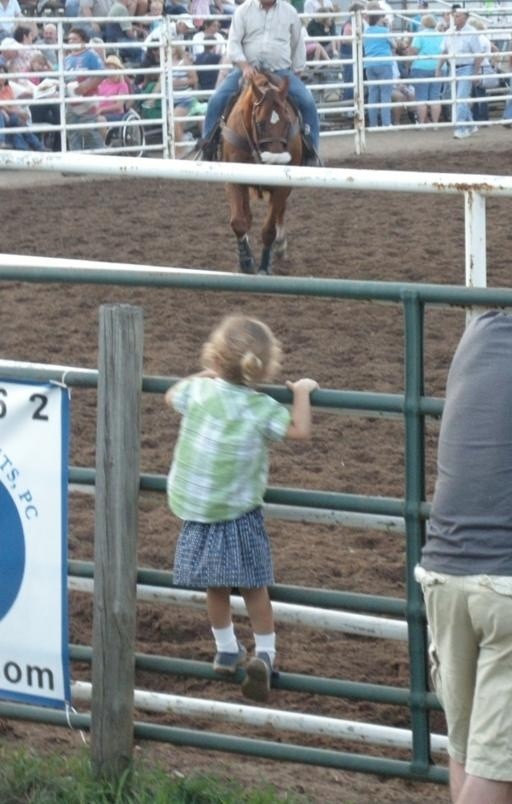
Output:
[241,651,273,703]
[212,639,249,676]
[452,126,479,140]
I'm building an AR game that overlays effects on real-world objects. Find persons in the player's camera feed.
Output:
[412,302,511,803]
[290,1,512,140]
[1,1,243,160]
[164,313,324,701]
[202,1,320,164]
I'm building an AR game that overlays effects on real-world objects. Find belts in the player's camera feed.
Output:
[456,64,474,69]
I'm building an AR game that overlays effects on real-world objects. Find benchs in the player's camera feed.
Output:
[0,0,512,151]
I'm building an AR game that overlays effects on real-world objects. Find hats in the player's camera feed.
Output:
[1,37,22,47]
[104,55,124,69]
[176,12,195,30]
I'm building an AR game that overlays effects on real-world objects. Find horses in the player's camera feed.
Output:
[220,72,305,277]
